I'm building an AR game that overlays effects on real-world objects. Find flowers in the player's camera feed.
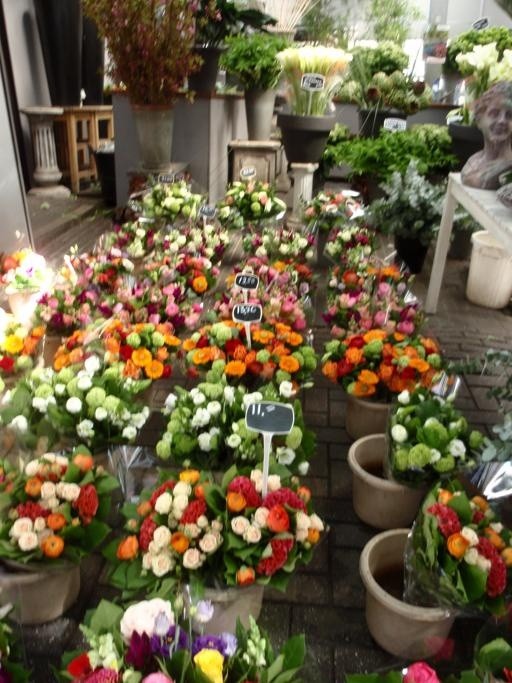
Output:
[35,265,100,332]
[0,356,151,452]
[100,266,203,332]
[320,266,452,399]
[446,41,512,125]
[277,46,353,116]
[101,462,222,596]
[218,175,282,225]
[349,38,424,115]
[404,482,512,620]
[343,637,512,683]
[211,256,317,330]
[0,248,52,295]
[321,245,371,290]
[68,246,134,296]
[242,223,314,262]
[304,189,360,226]
[62,600,307,683]
[132,174,204,223]
[155,372,317,475]
[0,317,47,381]
[144,215,230,295]
[359,155,471,247]
[53,315,180,381]
[99,219,156,259]
[179,319,318,387]
[385,386,489,487]
[323,224,382,263]
[0,447,119,565]
[208,458,328,592]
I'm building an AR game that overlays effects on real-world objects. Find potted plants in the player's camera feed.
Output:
[83,0,221,171]
[187,0,278,95]
[218,32,296,141]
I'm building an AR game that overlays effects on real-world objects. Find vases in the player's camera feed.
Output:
[347,433,422,530]
[5,271,53,319]
[182,581,264,635]
[275,112,336,164]
[344,393,390,441]
[359,109,407,138]
[216,197,286,261]
[0,560,80,624]
[447,121,485,171]
[129,251,155,277]
[357,527,455,660]
[392,232,430,274]
